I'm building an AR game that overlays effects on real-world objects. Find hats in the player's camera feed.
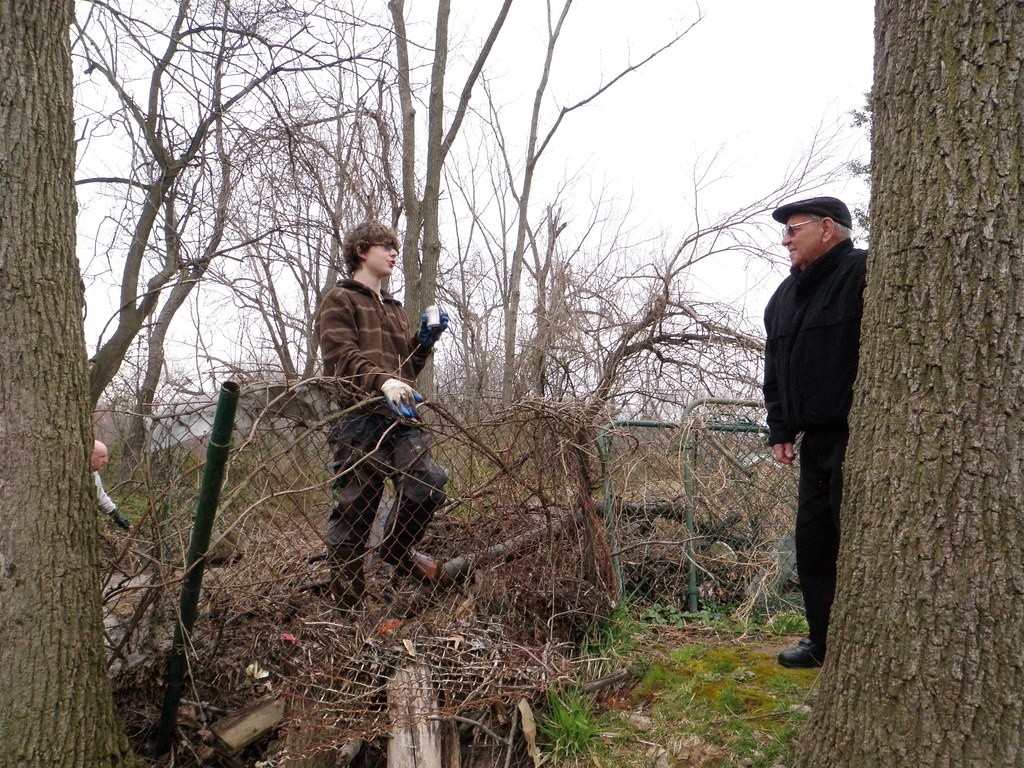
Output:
[772,196,852,231]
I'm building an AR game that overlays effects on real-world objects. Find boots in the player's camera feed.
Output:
[327,544,366,617]
[379,490,446,583]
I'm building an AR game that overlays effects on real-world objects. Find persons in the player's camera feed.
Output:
[762,197,870,667]
[314,220,448,621]
[90,440,133,534]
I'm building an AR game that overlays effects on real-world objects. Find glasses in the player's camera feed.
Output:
[782,220,816,237]
[366,242,400,255]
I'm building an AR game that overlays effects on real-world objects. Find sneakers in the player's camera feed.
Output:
[777,636,827,669]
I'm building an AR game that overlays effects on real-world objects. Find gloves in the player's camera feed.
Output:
[379,377,424,420]
[418,312,448,348]
